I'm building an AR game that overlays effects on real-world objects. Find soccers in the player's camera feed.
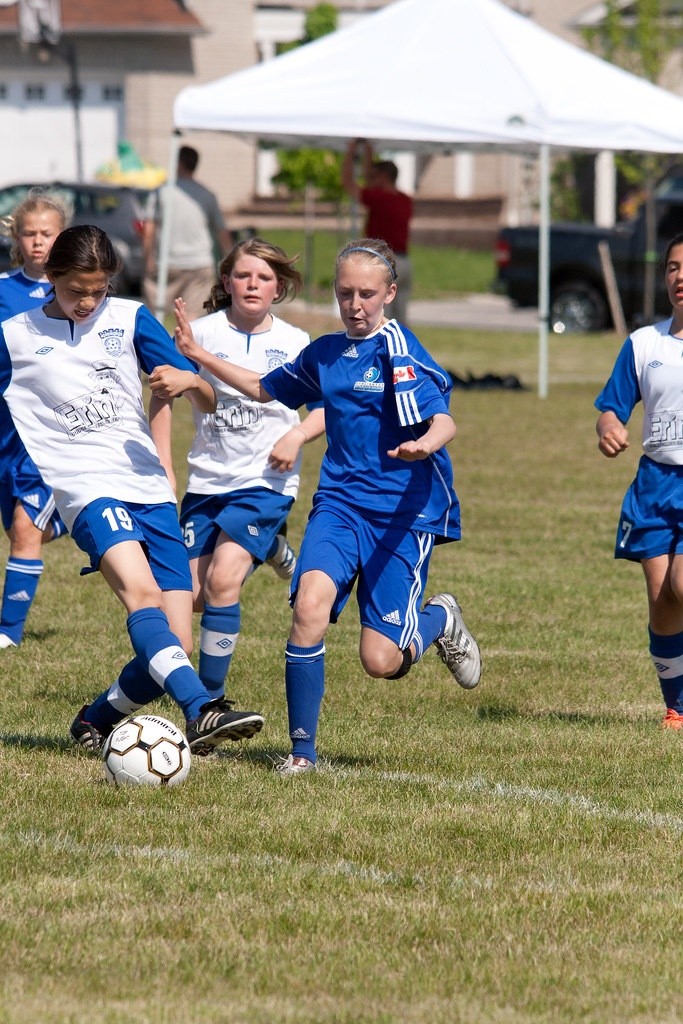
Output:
[102,713,199,794]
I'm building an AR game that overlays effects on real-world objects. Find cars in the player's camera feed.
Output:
[0,183,152,296]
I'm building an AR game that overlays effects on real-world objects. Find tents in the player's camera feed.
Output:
[153,0,683,399]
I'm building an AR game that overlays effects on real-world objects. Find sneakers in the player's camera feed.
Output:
[422,592,482,690]
[662,708,683,731]
[271,535,296,579]
[186,695,263,756]
[68,704,106,755]
[275,754,315,776]
[0,630,20,649]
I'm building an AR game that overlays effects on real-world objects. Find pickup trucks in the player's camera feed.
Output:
[497,163,682,335]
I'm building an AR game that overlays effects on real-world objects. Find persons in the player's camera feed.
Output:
[172,237,483,778]
[592,233,683,733]
[570,151,631,226]
[339,136,417,329]
[147,236,328,705]
[0,193,69,652]
[92,139,169,219]
[0,224,266,763]
[141,144,234,325]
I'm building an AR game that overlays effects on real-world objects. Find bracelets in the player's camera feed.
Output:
[294,425,308,443]
